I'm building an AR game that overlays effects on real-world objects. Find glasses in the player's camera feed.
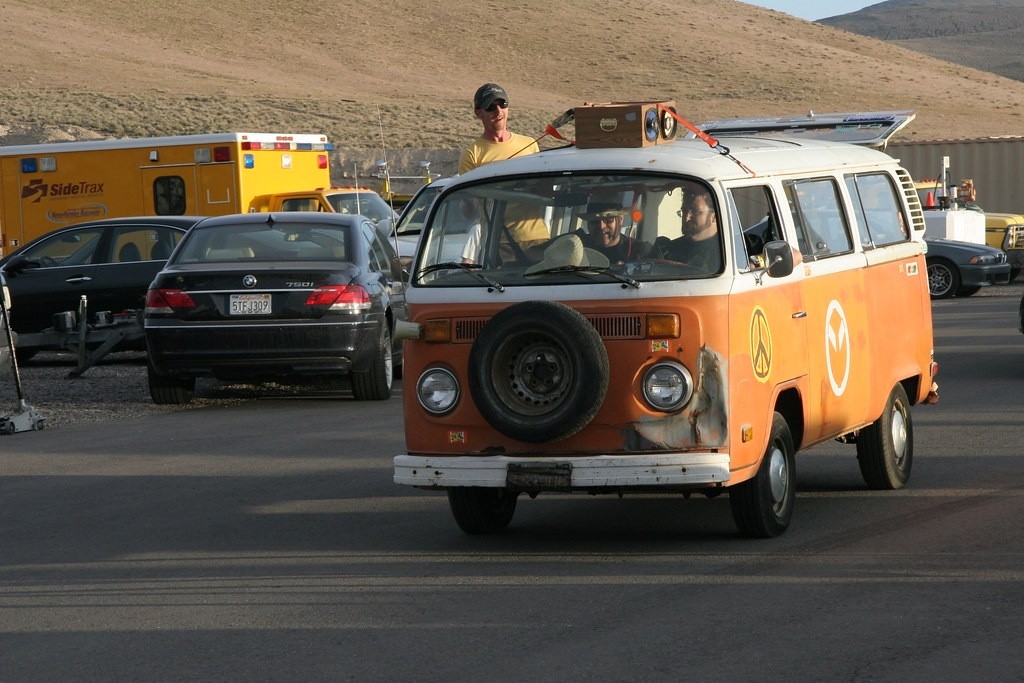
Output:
[677,209,716,217]
[588,216,620,226]
[486,100,508,112]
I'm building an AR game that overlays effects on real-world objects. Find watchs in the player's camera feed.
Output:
[750,256,760,268]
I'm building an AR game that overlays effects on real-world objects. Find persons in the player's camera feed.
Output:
[524,185,652,272]
[458,84,552,268]
[634,186,801,280]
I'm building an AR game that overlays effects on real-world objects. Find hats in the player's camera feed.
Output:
[574,188,631,220]
[525,235,610,280]
[474,84,508,109]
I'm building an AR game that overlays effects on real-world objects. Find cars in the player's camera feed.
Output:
[0,214,465,380]
[923,233,1011,297]
[143,209,411,410]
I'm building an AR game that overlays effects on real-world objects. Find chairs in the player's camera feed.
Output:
[119,242,141,262]
[151,241,172,260]
[203,247,255,259]
[297,246,336,258]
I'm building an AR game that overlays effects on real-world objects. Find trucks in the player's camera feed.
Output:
[0,131,406,280]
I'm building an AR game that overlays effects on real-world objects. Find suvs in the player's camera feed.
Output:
[872,182,1024,284]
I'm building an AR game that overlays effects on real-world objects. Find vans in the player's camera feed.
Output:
[385,99,939,539]
[386,174,586,295]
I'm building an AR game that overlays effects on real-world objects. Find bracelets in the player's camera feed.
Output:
[758,256,765,268]
